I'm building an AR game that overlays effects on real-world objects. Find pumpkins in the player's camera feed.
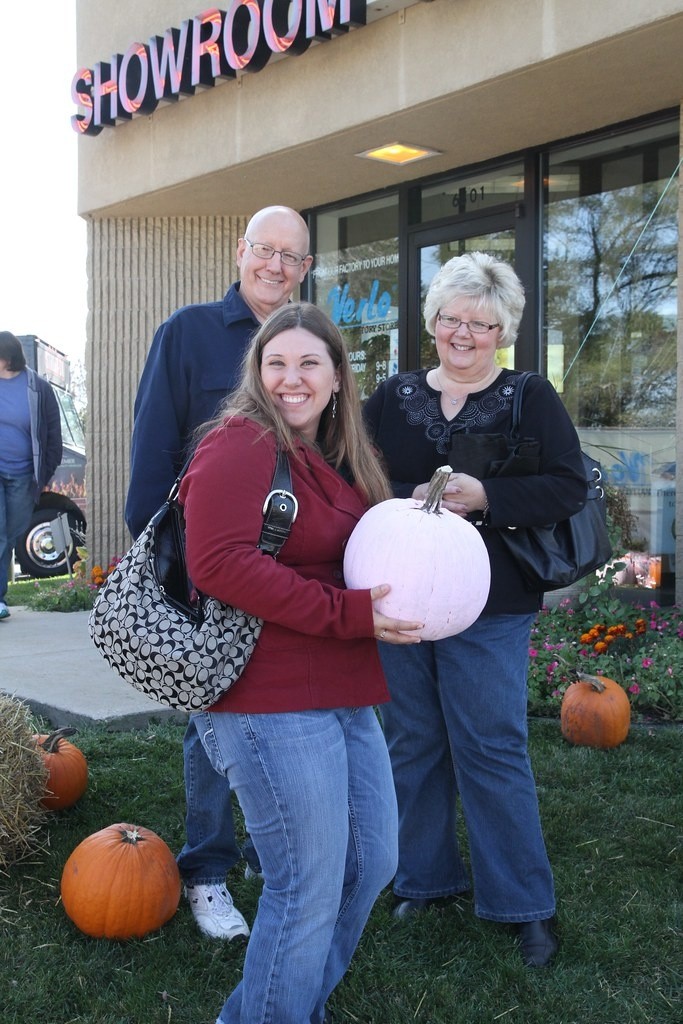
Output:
[60,822,180,941]
[344,466,490,642]
[562,671,631,747]
[30,726,89,810]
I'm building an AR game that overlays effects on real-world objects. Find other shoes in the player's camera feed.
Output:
[0,602,10,619]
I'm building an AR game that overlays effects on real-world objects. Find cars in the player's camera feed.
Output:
[14,445,88,579]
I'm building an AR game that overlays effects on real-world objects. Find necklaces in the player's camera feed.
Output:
[436,365,496,405]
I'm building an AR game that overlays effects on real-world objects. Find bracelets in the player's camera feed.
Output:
[484,498,490,518]
[380,629,387,639]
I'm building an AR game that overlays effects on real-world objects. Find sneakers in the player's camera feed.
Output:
[183,882,250,944]
[245,862,264,881]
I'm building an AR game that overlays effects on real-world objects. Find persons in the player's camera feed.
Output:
[179,302,423,1024]
[124,206,314,945]
[0,330,63,618]
[364,253,587,967]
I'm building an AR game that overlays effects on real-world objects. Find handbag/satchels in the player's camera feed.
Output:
[486,371,613,593]
[87,412,298,716]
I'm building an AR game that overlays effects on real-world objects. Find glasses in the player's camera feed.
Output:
[438,311,499,334]
[244,238,309,266]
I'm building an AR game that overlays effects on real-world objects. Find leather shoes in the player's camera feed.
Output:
[391,891,458,929]
[517,912,561,969]
